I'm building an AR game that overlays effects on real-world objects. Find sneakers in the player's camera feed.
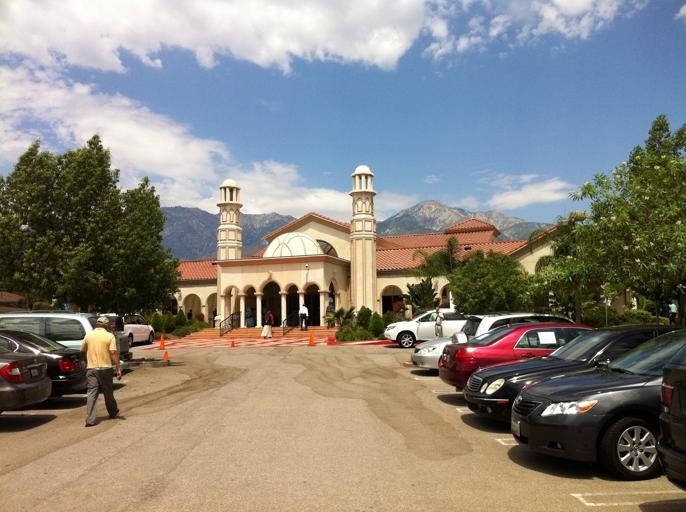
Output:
[85,408,119,427]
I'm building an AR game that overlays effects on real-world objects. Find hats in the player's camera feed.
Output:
[97,317,110,325]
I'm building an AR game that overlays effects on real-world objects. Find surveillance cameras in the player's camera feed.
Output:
[305,264,309,268]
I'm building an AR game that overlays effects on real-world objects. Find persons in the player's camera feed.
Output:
[326,303,335,329]
[246,306,255,328]
[79,316,123,428]
[298,303,309,330]
[667,303,679,325]
[429,308,446,336]
[212,308,216,327]
[261,309,275,339]
[187,309,192,319]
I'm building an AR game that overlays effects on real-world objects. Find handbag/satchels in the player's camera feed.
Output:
[264,317,271,325]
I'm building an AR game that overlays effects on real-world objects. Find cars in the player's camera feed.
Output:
[0,310,154,412]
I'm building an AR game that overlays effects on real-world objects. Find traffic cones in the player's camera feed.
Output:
[307,334,316,345]
[158,334,166,350]
[231,338,236,346]
[163,352,168,361]
